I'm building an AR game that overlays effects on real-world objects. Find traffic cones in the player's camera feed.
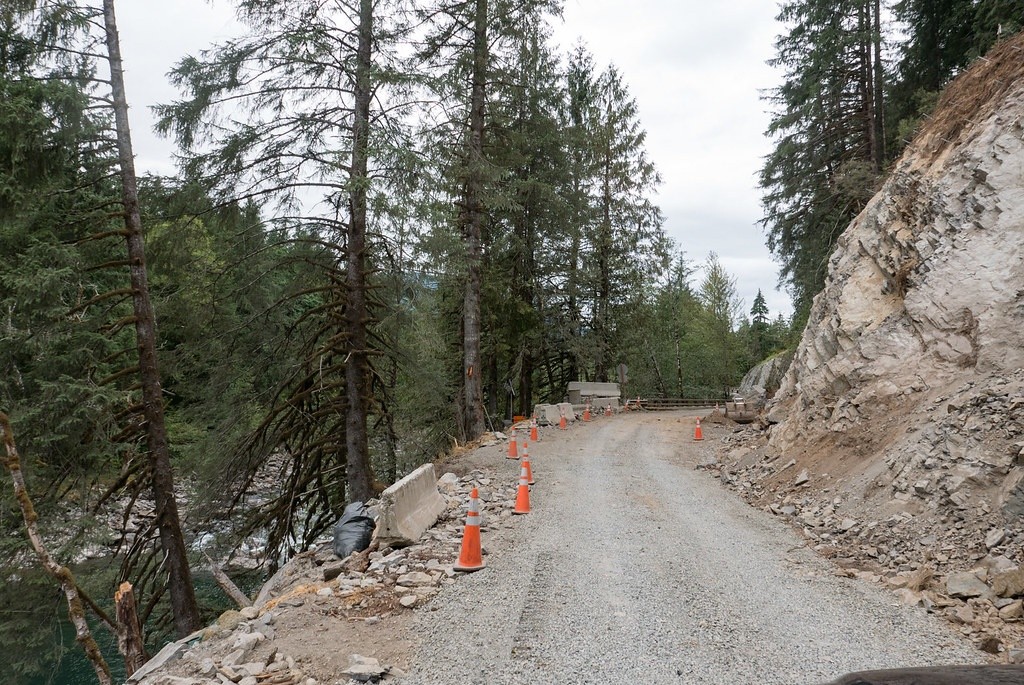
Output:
[582,401,591,422]
[510,458,532,515]
[529,415,540,442]
[623,401,629,413]
[635,396,641,411]
[452,487,487,572]
[505,425,520,460]
[692,416,705,441]
[604,402,612,416]
[558,407,569,430]
[517,440,535,485]
